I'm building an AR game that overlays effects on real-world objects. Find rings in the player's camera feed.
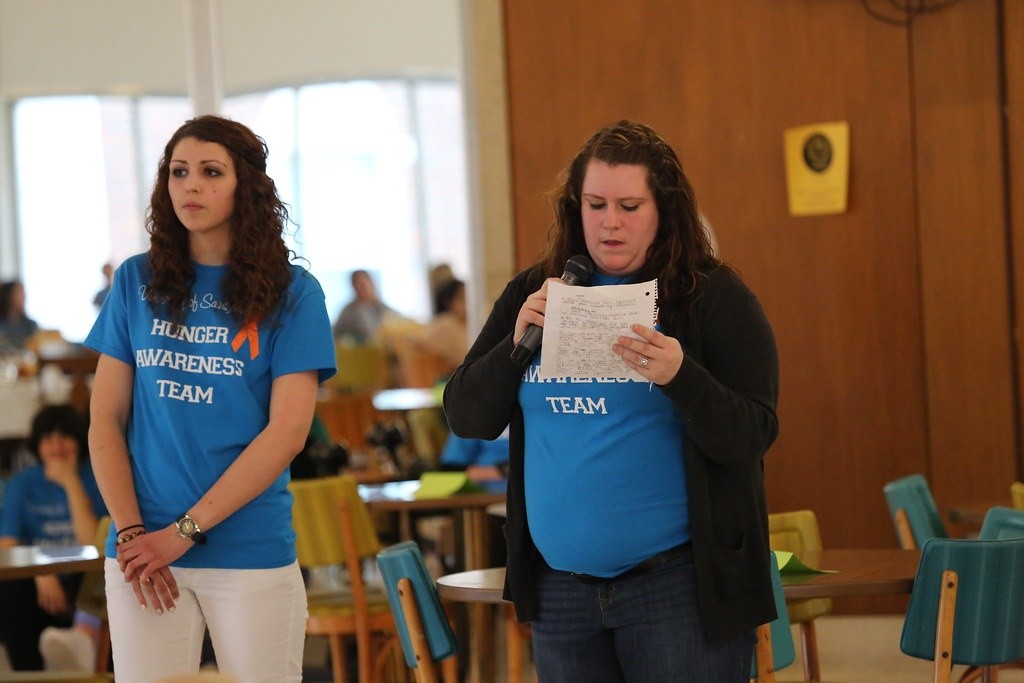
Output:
[147,578,151,581]
[640,357,648,368]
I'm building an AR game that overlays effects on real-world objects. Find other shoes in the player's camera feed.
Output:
[38,626,95,673]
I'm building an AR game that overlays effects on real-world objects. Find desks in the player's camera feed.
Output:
[435,549,924,682]
[356,478,506,682]
[36,342,100,409]
[370,388,441,411]
[0,540,105,581]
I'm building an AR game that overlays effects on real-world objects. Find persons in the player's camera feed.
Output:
[439,429,510,570]
[0,405,110,683]
[290,409,366,588]
[82,115,338,683]
[443,120,780,683]
[332,270,395,346]
[435,280,466,326]
[0,282,40,361]
[93,265,114,308]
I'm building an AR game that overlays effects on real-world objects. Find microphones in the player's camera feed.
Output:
[508,255,596,368]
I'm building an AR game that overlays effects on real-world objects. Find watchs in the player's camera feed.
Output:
[175,513,207,546]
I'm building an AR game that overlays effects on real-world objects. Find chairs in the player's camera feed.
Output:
[0,264,1024,683]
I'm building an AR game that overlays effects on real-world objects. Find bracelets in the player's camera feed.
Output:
[115,530,148,552]
[117,524,145,539]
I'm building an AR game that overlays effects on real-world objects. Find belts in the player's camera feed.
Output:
[568,540,690,584]
